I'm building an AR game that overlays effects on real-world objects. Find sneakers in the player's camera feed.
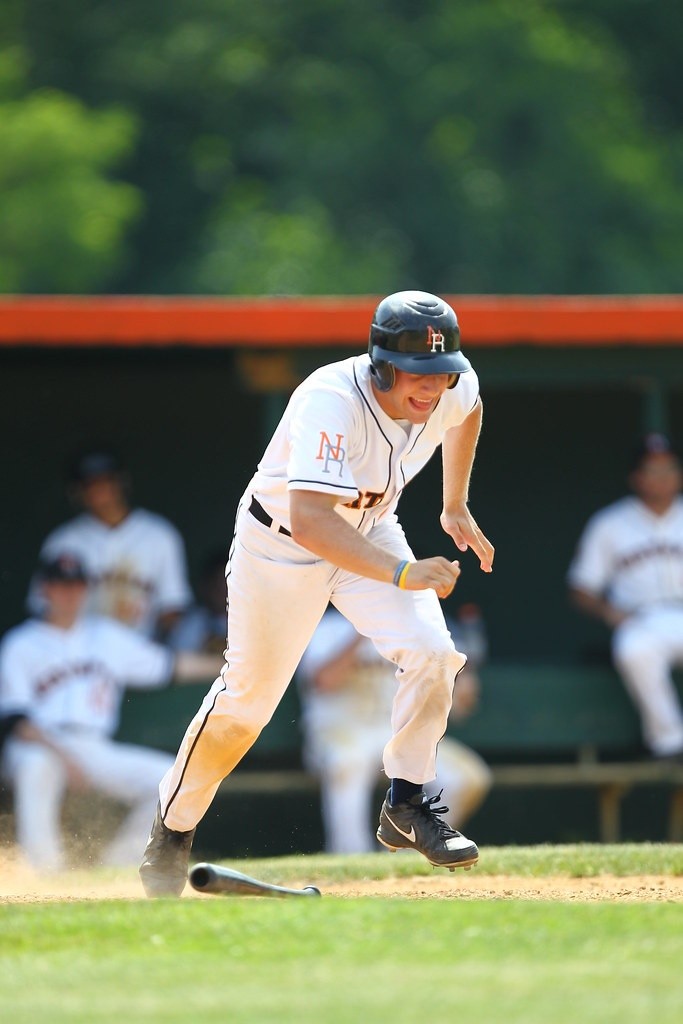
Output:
[375,786,479,873]
[140,798,196,898]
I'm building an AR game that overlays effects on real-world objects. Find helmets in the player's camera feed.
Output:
[369,290,473,392]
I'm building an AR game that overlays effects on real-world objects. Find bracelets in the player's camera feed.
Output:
[393,560,410,590]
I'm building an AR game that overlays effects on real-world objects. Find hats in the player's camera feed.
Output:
[42,558,85,583]
[76,460,120,490]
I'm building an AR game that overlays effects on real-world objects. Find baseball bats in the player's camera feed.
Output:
[187,860,321,900]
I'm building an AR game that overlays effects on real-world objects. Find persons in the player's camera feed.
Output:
[294,608,495,855]
[568,433,683,758]
[0,551,224,876]
[24,459,190,643]
[139,290,496,901]
